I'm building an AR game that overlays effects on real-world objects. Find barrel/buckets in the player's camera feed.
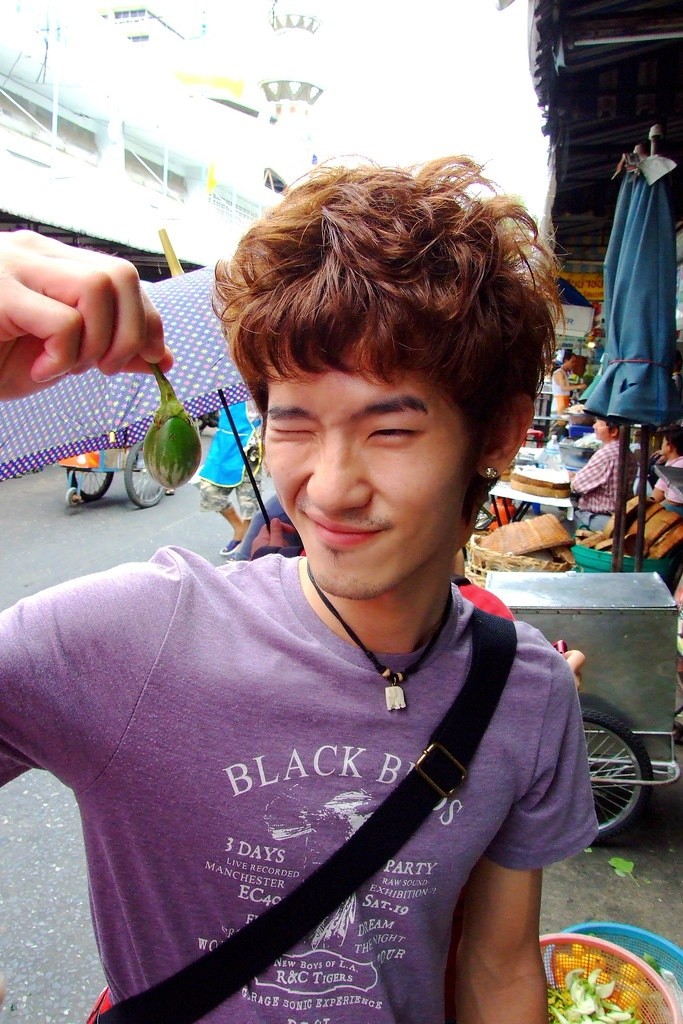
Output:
[571,543,677,596]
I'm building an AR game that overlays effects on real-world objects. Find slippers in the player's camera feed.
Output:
[220,540,241,555]
[226,553,237,563]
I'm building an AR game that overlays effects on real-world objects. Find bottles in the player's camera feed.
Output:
[545,435,559,469]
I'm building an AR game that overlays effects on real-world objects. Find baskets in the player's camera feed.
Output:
[466,568,486,589]
[543,923,683,1007]
[537,933,682,1024]
[468,535,569,576]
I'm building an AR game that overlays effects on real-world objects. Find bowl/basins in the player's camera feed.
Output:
[559,445,594,471]
[567,412,594,425]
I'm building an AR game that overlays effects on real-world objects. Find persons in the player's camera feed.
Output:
[547,353,588,442]
[200,397,265,557]
[672,350,683,394]
[245,519,585,695]
[0,155,599,1024]
[567,417,638,532]
[649,424,683,507]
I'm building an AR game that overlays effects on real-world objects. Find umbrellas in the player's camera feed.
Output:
[575,123,680,575]
[1,232,274,531]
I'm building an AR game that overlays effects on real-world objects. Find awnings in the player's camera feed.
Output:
[552,275,594,338]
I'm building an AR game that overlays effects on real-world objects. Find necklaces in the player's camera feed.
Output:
[307,561,452,711]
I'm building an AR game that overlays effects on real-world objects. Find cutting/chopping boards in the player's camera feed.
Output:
[510,470,570,498]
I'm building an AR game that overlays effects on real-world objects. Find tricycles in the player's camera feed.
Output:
[59,447,165,509]
[484,569,680,841]
